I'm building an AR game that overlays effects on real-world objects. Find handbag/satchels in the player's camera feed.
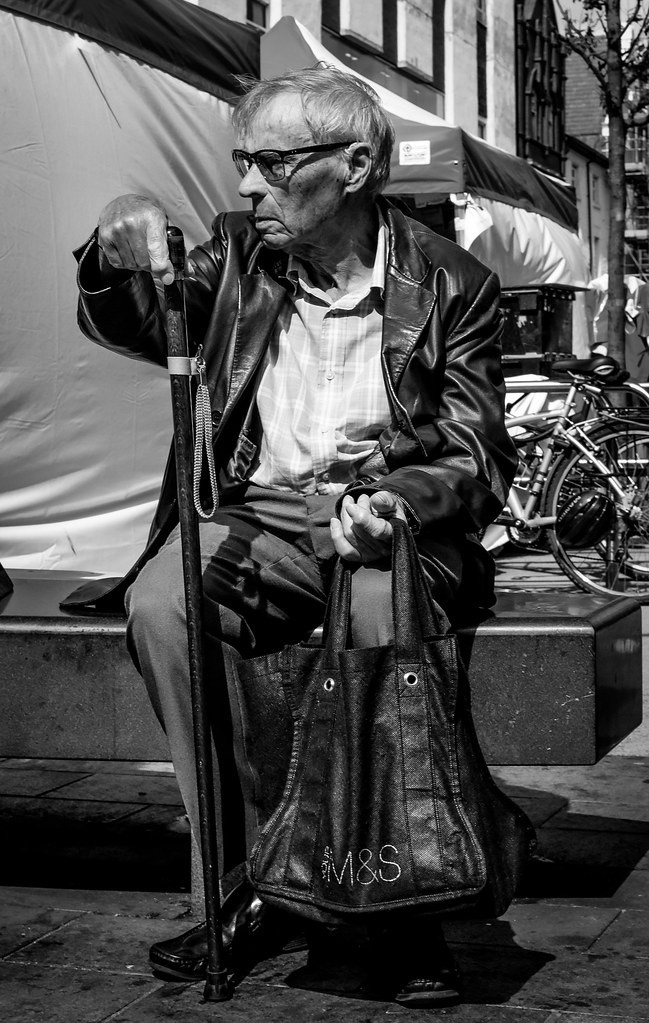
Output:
[231,516,540,936]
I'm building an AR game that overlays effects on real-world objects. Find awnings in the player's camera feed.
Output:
[258,22,580,251]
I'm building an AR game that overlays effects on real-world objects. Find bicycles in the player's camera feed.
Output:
[490,329,649,604]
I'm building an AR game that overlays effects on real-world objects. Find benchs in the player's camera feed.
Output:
[0,577,643,769]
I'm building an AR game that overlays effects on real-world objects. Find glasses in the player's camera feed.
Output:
[230,140,366,181]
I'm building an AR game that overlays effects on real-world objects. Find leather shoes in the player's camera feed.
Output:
[392,962,462,1010]
[147,849,284,984]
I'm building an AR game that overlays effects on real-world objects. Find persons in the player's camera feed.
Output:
[72,69,518,1003]
